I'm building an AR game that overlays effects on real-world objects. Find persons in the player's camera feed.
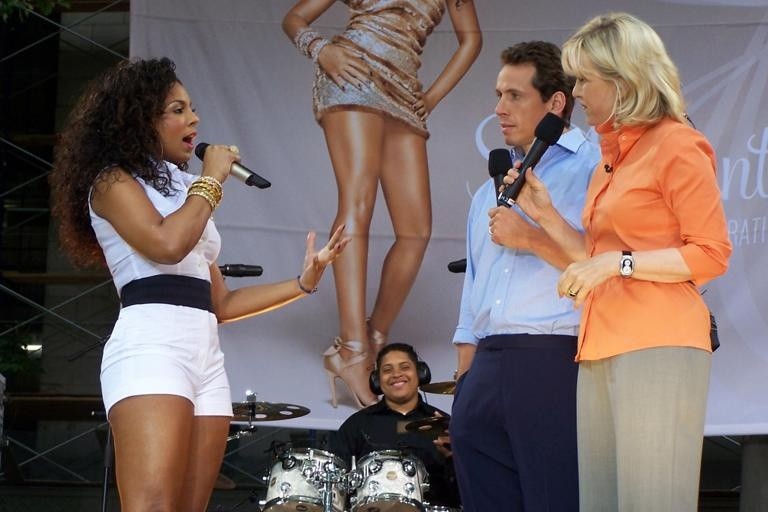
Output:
[448,38,603,512]
[281,0,484,410]
[332,342,466,510]
[499,13,735,512]
[51,57,353,512]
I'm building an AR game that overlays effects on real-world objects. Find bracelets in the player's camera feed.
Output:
[296,275,318,295]
[185,176,224,212]
[292,27,329,63]
[619,250,636,279]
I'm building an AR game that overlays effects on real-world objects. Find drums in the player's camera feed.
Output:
[351,450,431,510]
[259,446,347,512]
[427,504,464,512]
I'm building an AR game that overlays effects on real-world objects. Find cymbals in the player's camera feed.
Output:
[214,472,237,490]
[420,381,458,395]
[405,416,452,438]
[230,402,311,421]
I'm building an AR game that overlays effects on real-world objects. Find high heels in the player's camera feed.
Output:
[322,338,379,408]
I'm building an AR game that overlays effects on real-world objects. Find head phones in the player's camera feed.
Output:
[369,343,431,395]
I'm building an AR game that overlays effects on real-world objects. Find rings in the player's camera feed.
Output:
[569,288,577,297]
[489,226,493,235]
[228,145,239,154]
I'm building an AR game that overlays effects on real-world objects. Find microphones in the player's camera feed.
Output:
[489,149,513,206]
[224,263,263,277]
[195,142,272,188]
[448,258,466,273]
[497,111,565,209]
[604,164,613,173]
